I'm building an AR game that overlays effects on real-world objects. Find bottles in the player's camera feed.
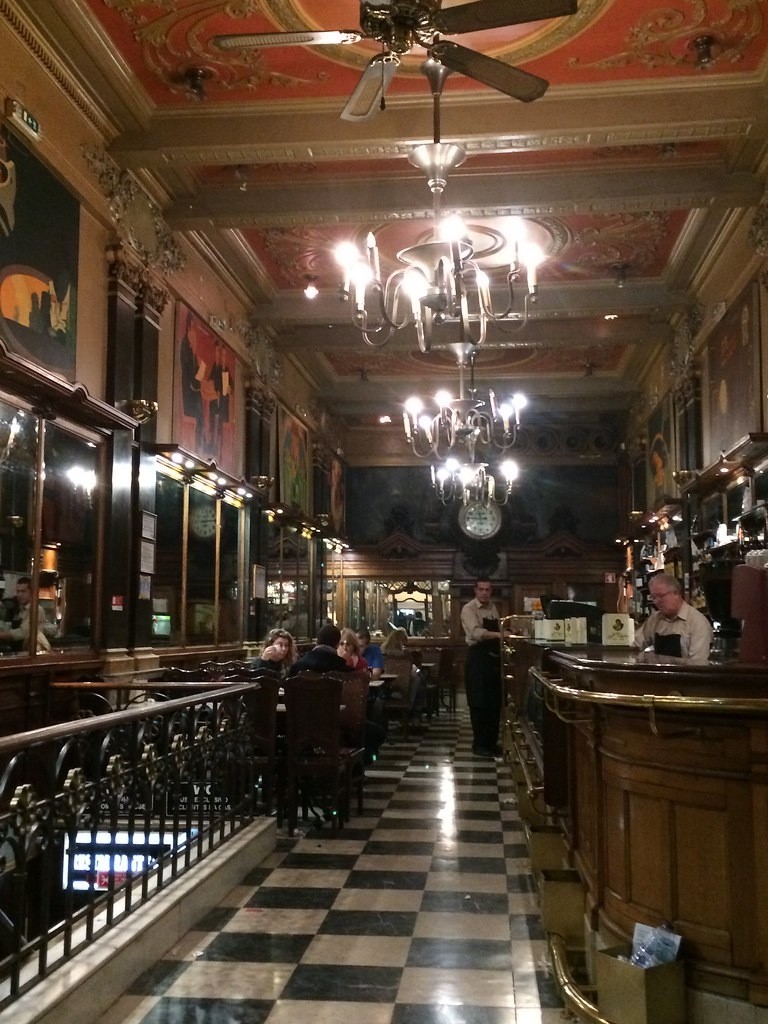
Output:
[631,924,673,969]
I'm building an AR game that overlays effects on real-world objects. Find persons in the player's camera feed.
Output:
[461,577,509,758]
[382,627,423,658]
[354,627,384,676]
[339,628,369,674]
[250,628,298,672]
[630,574,712,661]
[0,576,46,653]
[289,625,387,783]
[409,611,425,636]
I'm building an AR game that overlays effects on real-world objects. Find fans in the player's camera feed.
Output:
[211,0,581,124]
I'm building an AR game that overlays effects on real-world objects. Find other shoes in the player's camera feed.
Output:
[473,744,503,756]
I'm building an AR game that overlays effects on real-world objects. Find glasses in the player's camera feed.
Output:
[339,641,353,646]
[650,591,672,602]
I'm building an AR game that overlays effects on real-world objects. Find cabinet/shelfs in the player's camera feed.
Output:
[634,432,768,651]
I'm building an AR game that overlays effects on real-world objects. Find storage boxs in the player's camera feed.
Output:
[565,617,587,643]
[535,619,565,642]
[602,613,635,646]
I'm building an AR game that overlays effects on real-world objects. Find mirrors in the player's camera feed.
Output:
[1,385,344,662]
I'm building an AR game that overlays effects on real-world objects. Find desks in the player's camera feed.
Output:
[275,703,346,724]
[369,679,384,691]
[379,672,398,730]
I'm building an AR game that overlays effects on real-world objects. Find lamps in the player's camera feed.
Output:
[186,69,208,96]
[334,45,543,367]
[612,263,627,288]
[360,368,370,381]
[404,339,529,463]
[692,36,715,67]
[583,363,594,378]
[429,436,517,510]
[303,274,319,300]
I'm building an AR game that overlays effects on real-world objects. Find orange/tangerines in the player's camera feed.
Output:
[532,600,542,610]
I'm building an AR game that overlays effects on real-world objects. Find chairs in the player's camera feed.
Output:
[148,639,458,833]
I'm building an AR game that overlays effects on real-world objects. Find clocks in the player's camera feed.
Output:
[458,500,504,539]
